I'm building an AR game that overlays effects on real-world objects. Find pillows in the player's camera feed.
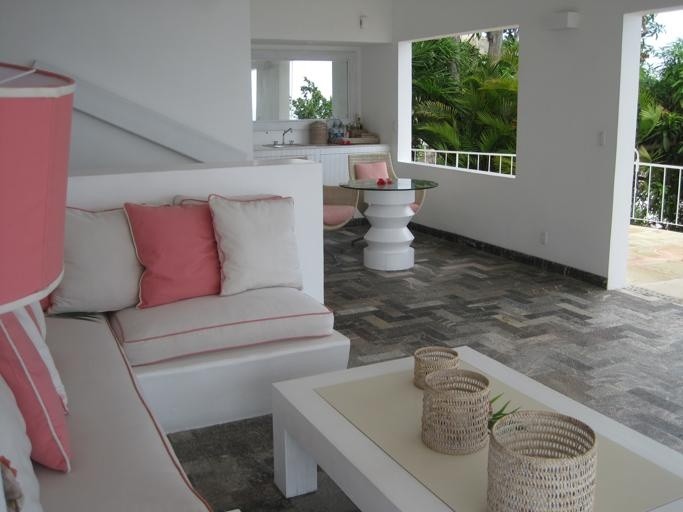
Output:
[0,304,81,512]
[122,199,222,309]
[45,204,146,318]
[323,204,353,226]
[207,190,304,296]
[354,160,389,180]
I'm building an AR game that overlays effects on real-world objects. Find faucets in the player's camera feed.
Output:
[282,128,292,144]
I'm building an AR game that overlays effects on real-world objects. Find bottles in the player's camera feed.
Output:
[328,117,364,146]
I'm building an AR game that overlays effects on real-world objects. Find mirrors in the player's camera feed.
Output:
[251,56,355,121]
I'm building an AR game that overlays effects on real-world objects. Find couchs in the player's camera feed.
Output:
[0,162,357,512]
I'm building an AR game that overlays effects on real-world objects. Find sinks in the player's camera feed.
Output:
[262,143,307,148]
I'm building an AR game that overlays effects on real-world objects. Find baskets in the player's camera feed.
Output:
[412,345,460,390]
[420,368,491,457]
[484,408,598,512]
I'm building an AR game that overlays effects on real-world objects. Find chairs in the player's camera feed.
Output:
[347,153,426,244]
[322,183,359,232]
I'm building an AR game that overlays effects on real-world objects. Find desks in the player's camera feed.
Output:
[339,178,439,273]
